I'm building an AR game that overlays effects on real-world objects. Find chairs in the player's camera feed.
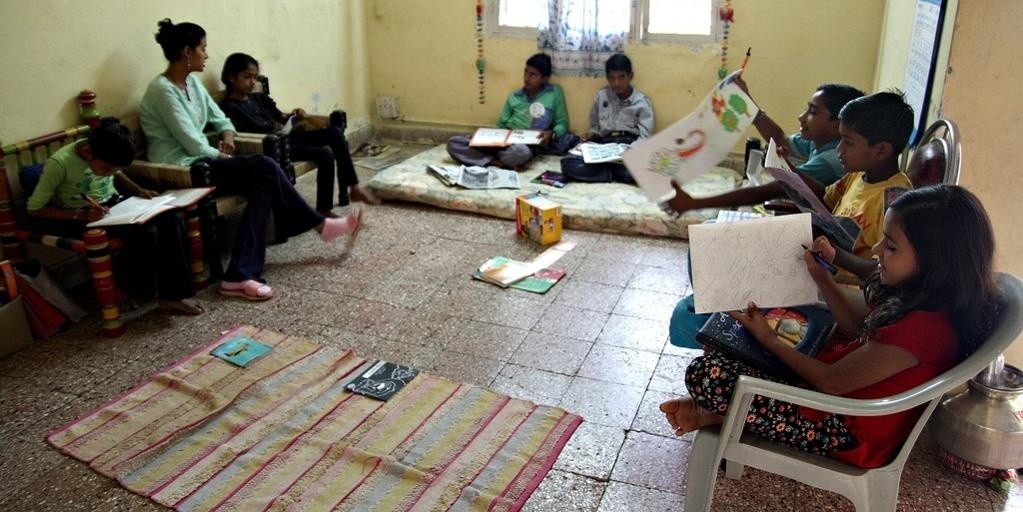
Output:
[908,117,965,195]
[683,267,1023,512]
[0,70,349,337]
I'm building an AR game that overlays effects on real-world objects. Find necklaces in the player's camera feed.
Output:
[165,68,187,92]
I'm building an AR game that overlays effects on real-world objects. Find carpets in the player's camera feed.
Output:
[46,321,590,512]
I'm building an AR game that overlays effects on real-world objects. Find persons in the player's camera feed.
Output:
[137,16,364,303]
[217,51,381,219]
[657,181,1003,472]
[442,52,569,170]
[21,116,207,318]
[669,89,915,349]
[657,73,866,295]
[584,54,655,141]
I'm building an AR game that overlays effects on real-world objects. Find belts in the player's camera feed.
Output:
[602,129,639,139]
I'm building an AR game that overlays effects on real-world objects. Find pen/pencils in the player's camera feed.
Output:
[380,145,390,153]
[801,244,838,275]
[738,47,751,79]
[79,192,110,215]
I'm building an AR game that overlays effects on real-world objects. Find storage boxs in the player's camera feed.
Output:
[512,191,564,247]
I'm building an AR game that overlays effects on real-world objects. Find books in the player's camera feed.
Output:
[348,140,404,171]
[424,162,521,190]
[567,135,645,166]
[468,127,544,148]
[762,136,856,253]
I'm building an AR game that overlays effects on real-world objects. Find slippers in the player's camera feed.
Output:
[217,282,273,301]
[344,202,364,250]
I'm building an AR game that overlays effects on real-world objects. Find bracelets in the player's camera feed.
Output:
[751,108,766,125]
[72,207,80,220]
